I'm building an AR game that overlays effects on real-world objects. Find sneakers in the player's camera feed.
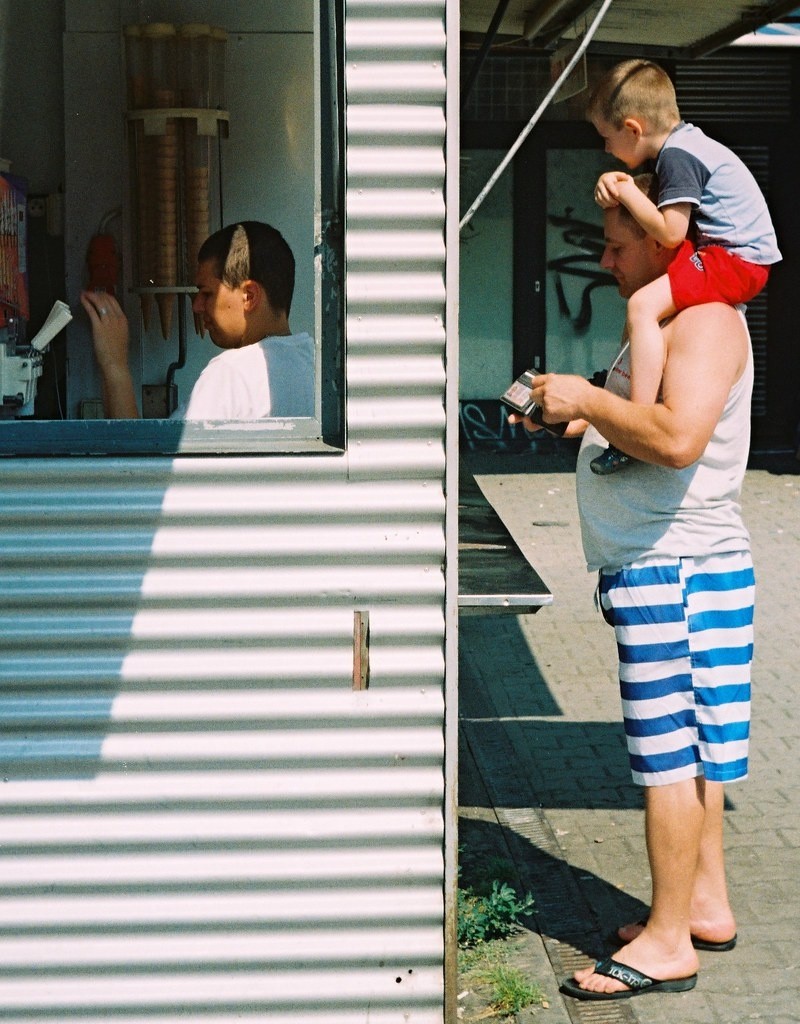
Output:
[590,443,641,475]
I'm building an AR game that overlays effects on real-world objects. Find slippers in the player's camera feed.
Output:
[560,914,738,1001]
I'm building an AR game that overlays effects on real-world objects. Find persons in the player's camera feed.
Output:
[79,219,318,420]
[581,56,782,473]
[499,169,762,997]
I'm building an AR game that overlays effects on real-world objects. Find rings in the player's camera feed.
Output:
[99,309,108,315]
